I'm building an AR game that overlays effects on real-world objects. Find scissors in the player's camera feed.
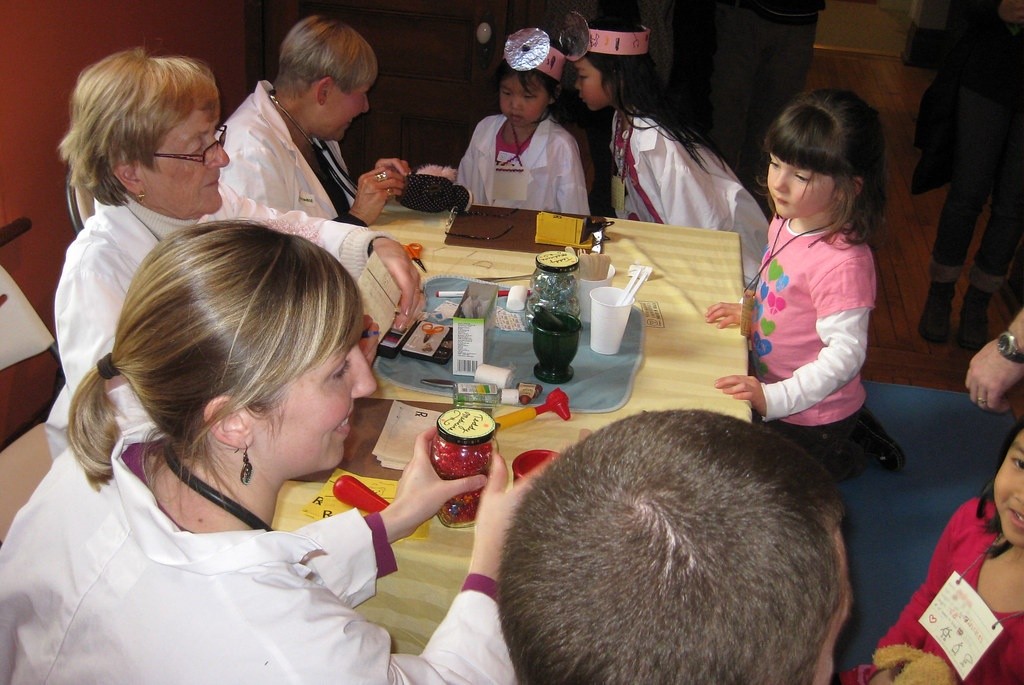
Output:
[421,323,445,343]
[402,243,427,274]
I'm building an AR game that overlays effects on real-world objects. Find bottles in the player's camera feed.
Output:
[524,250,583,337]
[431,407,500,528]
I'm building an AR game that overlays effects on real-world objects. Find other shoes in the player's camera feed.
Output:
[854,407,906,471]
[959,281,993,349]
[920,278,955,343]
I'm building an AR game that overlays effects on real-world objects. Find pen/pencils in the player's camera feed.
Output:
[360,329,381,339]
[435,290,510,298]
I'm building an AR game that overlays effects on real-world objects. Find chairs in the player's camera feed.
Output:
[0,217,65,544]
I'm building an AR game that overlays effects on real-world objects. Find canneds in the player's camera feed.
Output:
[431,408,496,528]
[527,251,581,320]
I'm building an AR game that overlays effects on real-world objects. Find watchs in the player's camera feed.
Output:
[997,331,1024,363]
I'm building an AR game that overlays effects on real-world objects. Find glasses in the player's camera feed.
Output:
[154,123,227,166]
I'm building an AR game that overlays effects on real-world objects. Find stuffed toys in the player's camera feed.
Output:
[872,645,955,685]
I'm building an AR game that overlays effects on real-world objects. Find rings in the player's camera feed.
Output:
[415,288,421,292]
[375,171,389,182]
[387,188,394,197]
[977,397,987,403]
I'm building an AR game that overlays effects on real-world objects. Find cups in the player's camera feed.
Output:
[512,450,559,484]
[531,312,582,385]
[588,285,635,354]
[579,265,616,323]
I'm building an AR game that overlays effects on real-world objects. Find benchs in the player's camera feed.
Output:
[830,381,1018,685]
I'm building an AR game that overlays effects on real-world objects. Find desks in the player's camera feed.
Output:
[272,196,752,655]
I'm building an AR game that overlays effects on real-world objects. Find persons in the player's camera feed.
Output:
[215,15,411,228]
[500,410,853,685]
[0,218,592,685]
[703,88,906,477]
[559,13,769,293]
[47,47,427,460]
[540,0,1024,413]
[453,28,591,218]
[832,414,1024,685]
